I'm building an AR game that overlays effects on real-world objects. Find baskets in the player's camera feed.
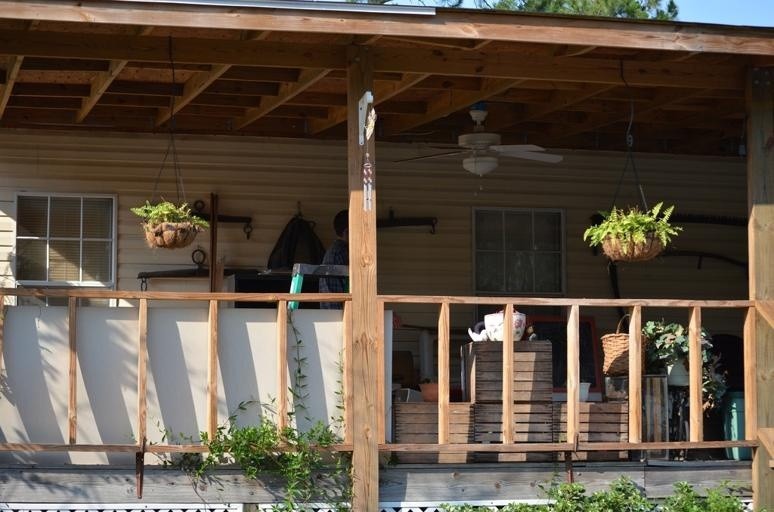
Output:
[602,232,662,261]
[600,314,647,377]
[141,222,200,247]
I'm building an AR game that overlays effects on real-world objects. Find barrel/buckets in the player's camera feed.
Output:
[723,392,752,460]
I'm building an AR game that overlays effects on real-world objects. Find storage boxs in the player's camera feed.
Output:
[391,338,670,462]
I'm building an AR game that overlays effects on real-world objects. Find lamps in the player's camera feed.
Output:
[463,156,498,177]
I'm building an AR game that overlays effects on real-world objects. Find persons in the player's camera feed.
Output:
[318,210,401,329]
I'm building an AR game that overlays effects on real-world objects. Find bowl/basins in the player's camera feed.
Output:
[483,313,527,342]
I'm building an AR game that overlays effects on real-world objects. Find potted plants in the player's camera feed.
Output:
[132,201,209,248]
[581,204,681,263]
[643,320,713,388]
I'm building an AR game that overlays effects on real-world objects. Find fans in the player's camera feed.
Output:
[393,102,564,165]
